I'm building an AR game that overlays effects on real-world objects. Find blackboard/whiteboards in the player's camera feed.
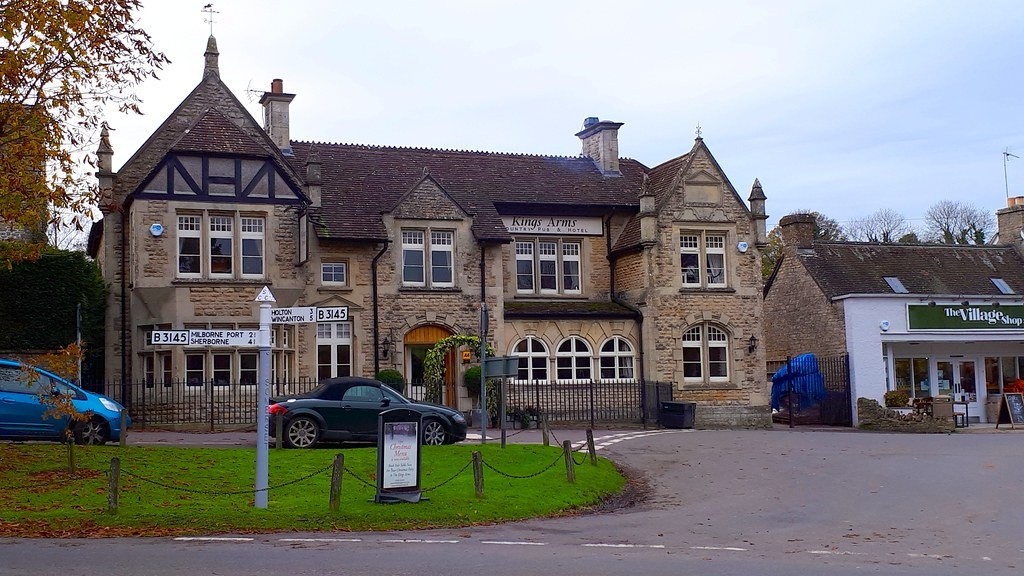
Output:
[997,393,1024,423]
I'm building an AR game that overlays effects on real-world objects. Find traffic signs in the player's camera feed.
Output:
[259,306,349,325]
[152,328,272,348]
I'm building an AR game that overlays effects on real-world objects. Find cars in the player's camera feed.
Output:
[266,376,467,445]
[0,359,132,447]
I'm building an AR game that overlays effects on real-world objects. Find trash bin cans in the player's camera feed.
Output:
[933,395,955,431]
[660,401,697,429]
